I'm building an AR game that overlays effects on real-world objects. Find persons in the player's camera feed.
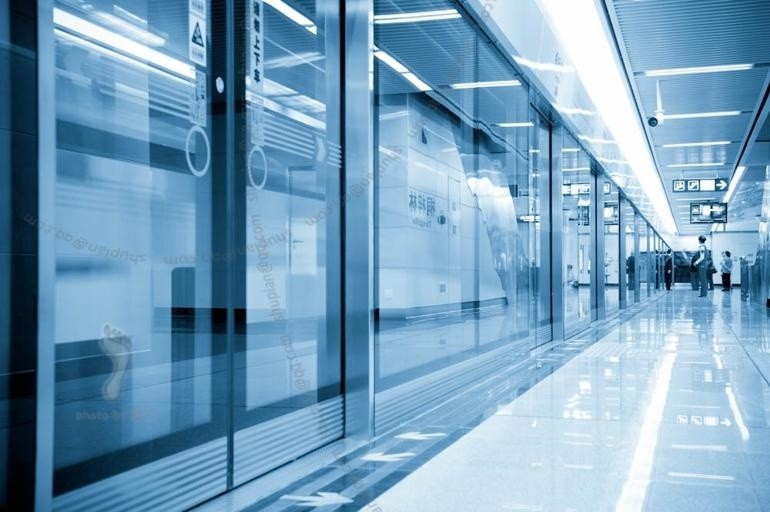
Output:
[652,248,660,291]
[720,249,733,291]
[689,250,700,291]
[708,248,718,290]
[720,251,725,267]
[626,250,637,291]
[693,235,710,298]
[665,248,673,291]
